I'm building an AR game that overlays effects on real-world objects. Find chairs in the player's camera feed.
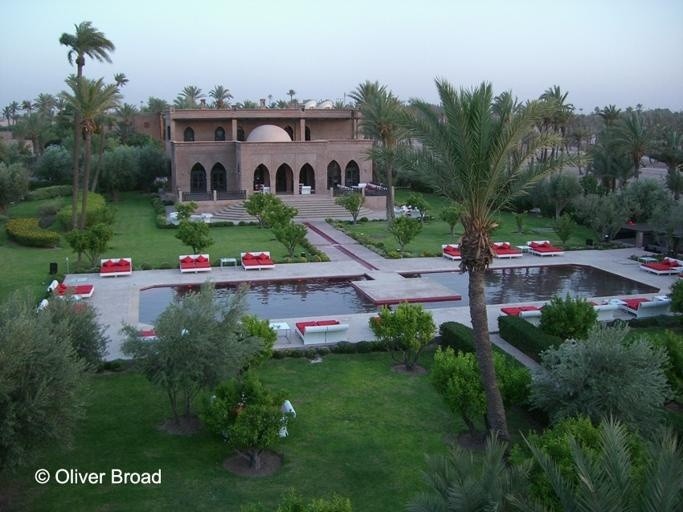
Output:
[38,249,349,347]
[441,240,683,327]
